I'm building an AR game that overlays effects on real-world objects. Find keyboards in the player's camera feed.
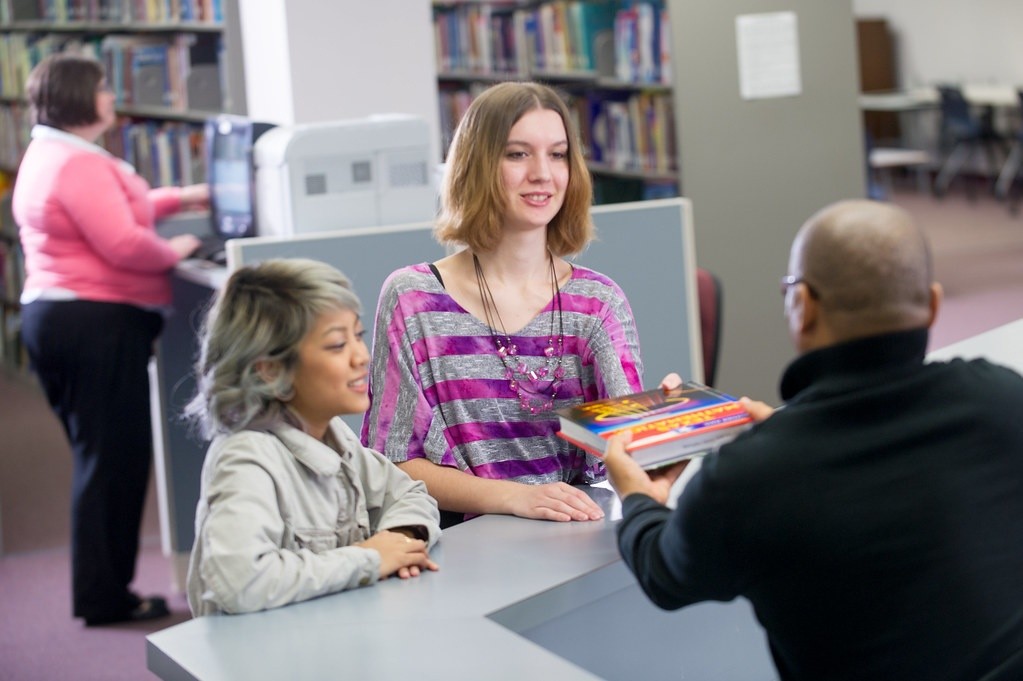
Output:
[190,234,226,259]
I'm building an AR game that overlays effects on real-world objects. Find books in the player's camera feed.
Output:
[1,104,34,372]
[433,0,670,84]
[554,381,755,469]
[0,0,224,20]
[92,118,205,188]
[439,85,488,158]
[560,88,676,197]
[0,31,221,110]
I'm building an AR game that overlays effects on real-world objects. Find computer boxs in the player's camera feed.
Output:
[255,113,438,239]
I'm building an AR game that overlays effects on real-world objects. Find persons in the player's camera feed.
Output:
[361,83,683,529]
[601,202,1023,681]
[185,257,443,619]
[10,56,210,625]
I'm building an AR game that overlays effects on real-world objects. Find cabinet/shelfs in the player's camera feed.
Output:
[0,0,866,413]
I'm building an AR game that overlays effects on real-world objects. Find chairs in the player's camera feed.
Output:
[859,81,1023,200]
[694,265,723,391]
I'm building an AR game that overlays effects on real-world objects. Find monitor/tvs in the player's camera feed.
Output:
[205,116,285,241]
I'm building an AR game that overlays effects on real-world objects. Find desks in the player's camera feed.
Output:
[148,196,703,585]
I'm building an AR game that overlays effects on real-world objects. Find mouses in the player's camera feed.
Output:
[213,248,227,264]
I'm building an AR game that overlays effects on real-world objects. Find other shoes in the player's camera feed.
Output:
[86,589,169,627]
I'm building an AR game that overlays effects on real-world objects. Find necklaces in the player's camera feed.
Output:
[472,248,564,413]
